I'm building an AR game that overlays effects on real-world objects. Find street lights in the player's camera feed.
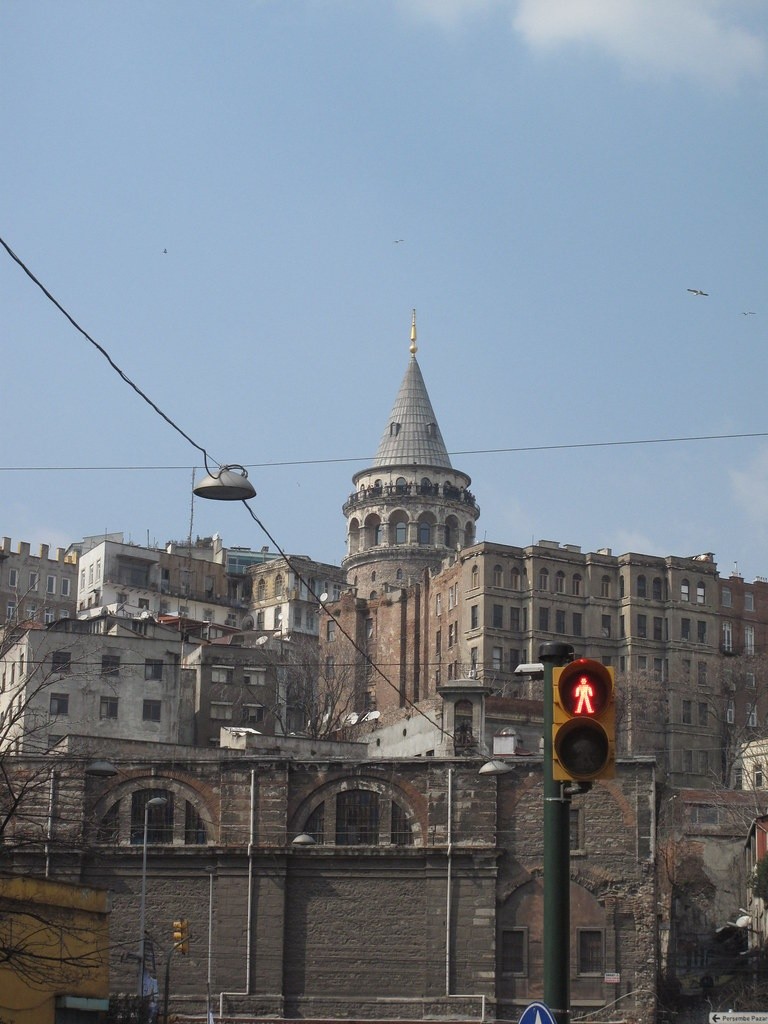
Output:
[136,797,169,996]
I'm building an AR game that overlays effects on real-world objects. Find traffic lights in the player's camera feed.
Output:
[552,657,618,781]
[173,919,189,955]
